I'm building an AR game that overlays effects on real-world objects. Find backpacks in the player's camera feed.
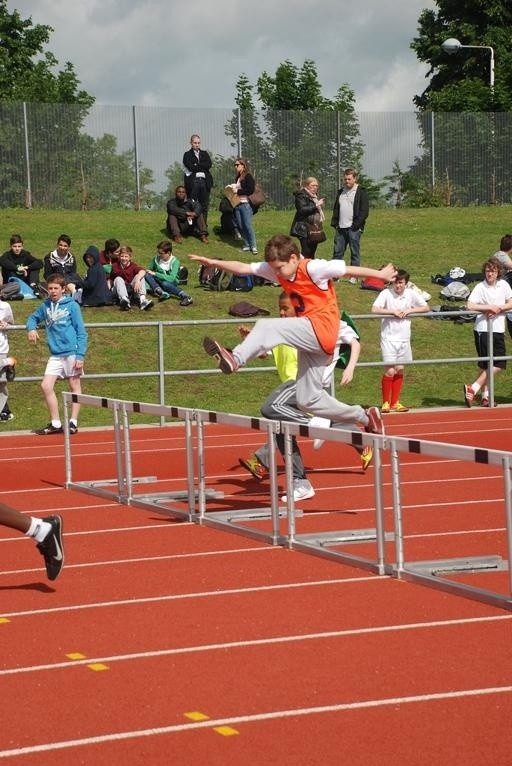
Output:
[197,257,280,292]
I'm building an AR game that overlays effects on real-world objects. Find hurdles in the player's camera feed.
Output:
[60,390,214,506]
[282,419,501,576]
[119,399,288,524]
[192,407,376,548]
[384,436,512,614]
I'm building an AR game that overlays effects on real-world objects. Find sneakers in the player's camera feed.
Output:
[158,291,170,302]
[307,393,409,470]
[281,478,316,502]
[181,295,193,305]
[481,399,497,407]
[1,357,77,434]
[464,384,474,407]
[37,514,65,581]
[174,234,258,255]
[203,335,238,374]
[140,300,154,310]
[120,296,132,311]
[11,294,24,300]
[239,456,267,480]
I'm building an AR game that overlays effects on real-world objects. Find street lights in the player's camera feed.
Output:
[441,37,499,203]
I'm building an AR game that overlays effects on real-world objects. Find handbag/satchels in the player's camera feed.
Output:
[228,302,269,318]
[440,282,470,301]
[360,278,385,291]
[34,281,49,298]
[306,221,326,245]
[179,265,188,285]
[248,183,266,206]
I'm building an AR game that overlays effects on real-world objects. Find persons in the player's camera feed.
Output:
[238,320,361,480]
[187,233,398,450]
[1,301,17,422]
[463,255,512,407]
[493,234,512,287]
[370,269,430,412]
[239,289,331,503]
[219,159,258,255]
[1,502,64,581]
[330,169,368,284]
[167,186,210,244]
[1,234,193,313]
[289,177,325,259]
[26,273,89,435]
[183,134,213,227]
